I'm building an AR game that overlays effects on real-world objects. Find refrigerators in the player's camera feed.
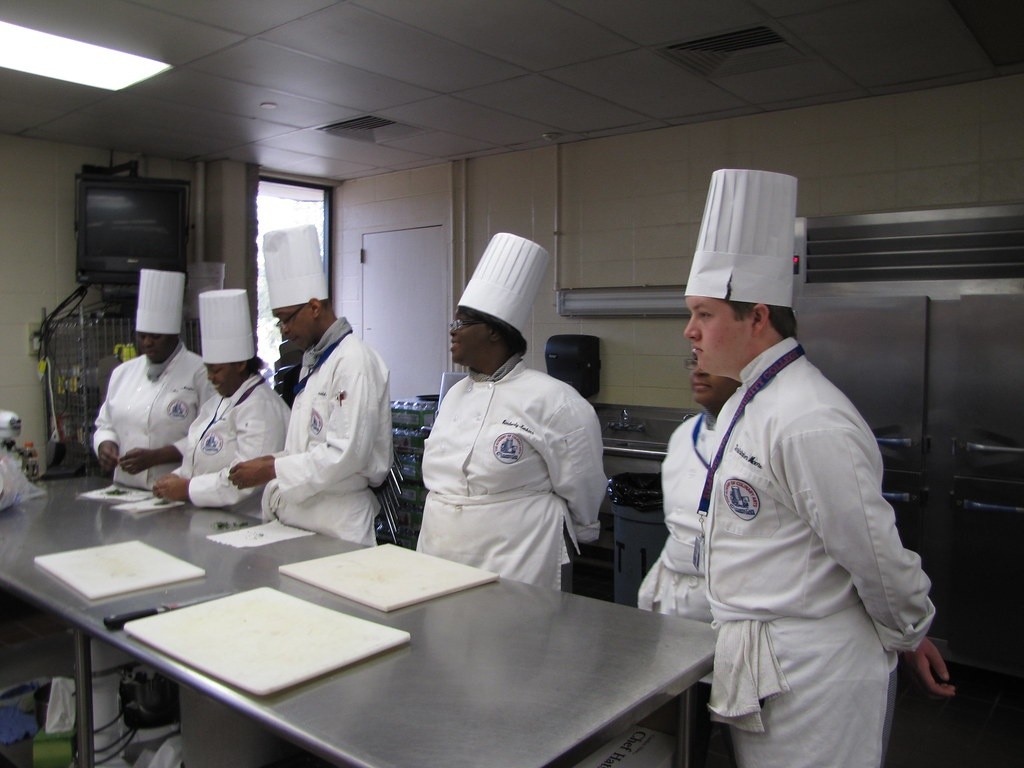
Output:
[790,204,1024,677]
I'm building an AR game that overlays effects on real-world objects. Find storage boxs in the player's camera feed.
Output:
[573,725,677,768]
[375,401,438,549]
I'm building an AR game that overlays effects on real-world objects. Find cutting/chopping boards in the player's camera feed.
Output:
[123,585,412,696]
[34,541,205,601]
[279,543,500,613]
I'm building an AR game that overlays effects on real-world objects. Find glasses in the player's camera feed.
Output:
[276,306,304,330]
[686,359,697,370]
[449,319,485,330]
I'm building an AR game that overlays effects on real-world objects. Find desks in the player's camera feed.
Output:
[0,477,723,768]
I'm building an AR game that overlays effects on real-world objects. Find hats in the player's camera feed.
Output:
[136,269,186,335]
[684,168,798,309]
[198,288,256,365]
[263,225,328,310]
[457,233,550,334]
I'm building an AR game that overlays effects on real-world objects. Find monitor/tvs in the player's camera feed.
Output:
[77,181,187,275]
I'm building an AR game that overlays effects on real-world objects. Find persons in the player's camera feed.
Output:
[227,222,395,546]
[92,268,221,768]
[412,230,610,594]
[152,288,293,768]
[636,345,743,768]
[682,167,957,768]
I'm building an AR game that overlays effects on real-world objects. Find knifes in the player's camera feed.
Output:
[102,592,228,631]
